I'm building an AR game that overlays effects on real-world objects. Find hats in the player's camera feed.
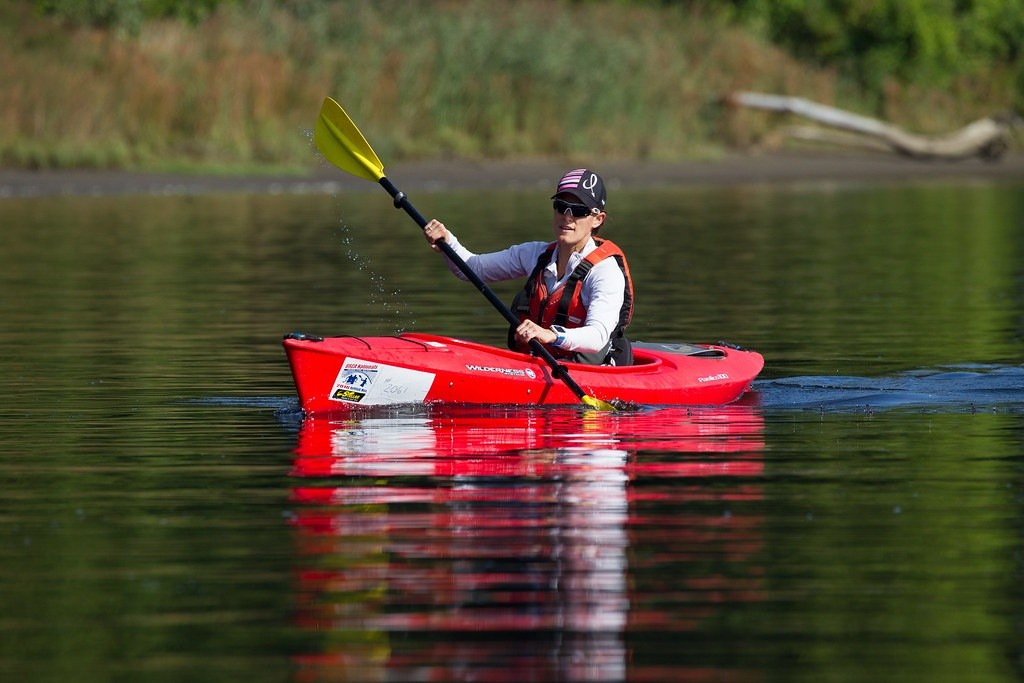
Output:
[550,168,607,213]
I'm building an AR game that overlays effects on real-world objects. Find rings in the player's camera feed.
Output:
[525,330,530,337]
[428,227,432,231]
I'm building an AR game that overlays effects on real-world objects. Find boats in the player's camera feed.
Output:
[285,333,764,417]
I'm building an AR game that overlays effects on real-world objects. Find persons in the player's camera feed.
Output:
[425,169,634,366]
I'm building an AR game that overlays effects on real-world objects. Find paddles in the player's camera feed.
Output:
[310,94,621,414]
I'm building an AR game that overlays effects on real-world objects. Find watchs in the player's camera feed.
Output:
[549,325,566,347]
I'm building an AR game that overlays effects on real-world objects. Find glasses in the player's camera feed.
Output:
[553,197,591,217]
